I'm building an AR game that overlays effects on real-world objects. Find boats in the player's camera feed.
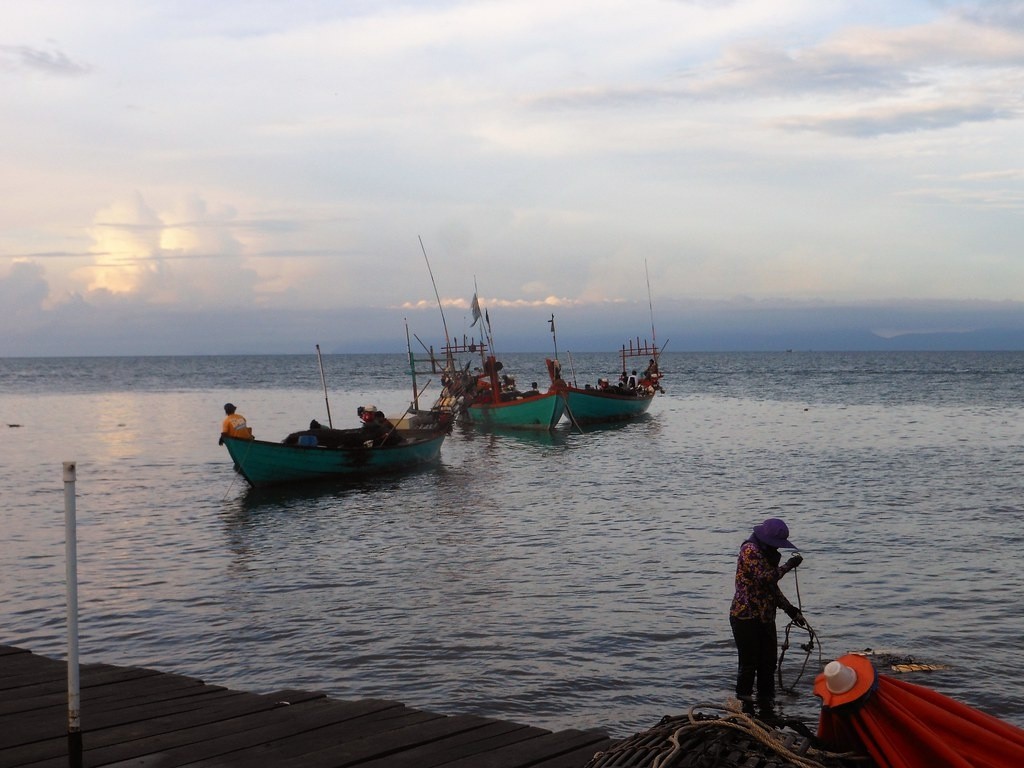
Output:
[418,233,570,431]
[548,258,670,423]
[219,314,456,497]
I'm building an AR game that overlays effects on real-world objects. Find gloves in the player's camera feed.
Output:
[784,606,806,628]
[787,555,803,568]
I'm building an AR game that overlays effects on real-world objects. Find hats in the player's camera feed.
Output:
[364,406,377,412]
[754,518,797,548]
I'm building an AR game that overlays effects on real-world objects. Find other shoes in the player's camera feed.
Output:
[741,701,755,715]
[758,707,787,720]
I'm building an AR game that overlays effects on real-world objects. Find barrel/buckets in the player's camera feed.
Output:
[298,436,317,447]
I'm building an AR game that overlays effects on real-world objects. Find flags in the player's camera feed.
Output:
[468,295,480,327]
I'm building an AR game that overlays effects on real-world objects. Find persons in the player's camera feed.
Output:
[475,367,539,396]
[357,404,399,446]
[585,370,638,392]
[730,518,807,728]
[218,403,253,446]
[643,358,659,376]
